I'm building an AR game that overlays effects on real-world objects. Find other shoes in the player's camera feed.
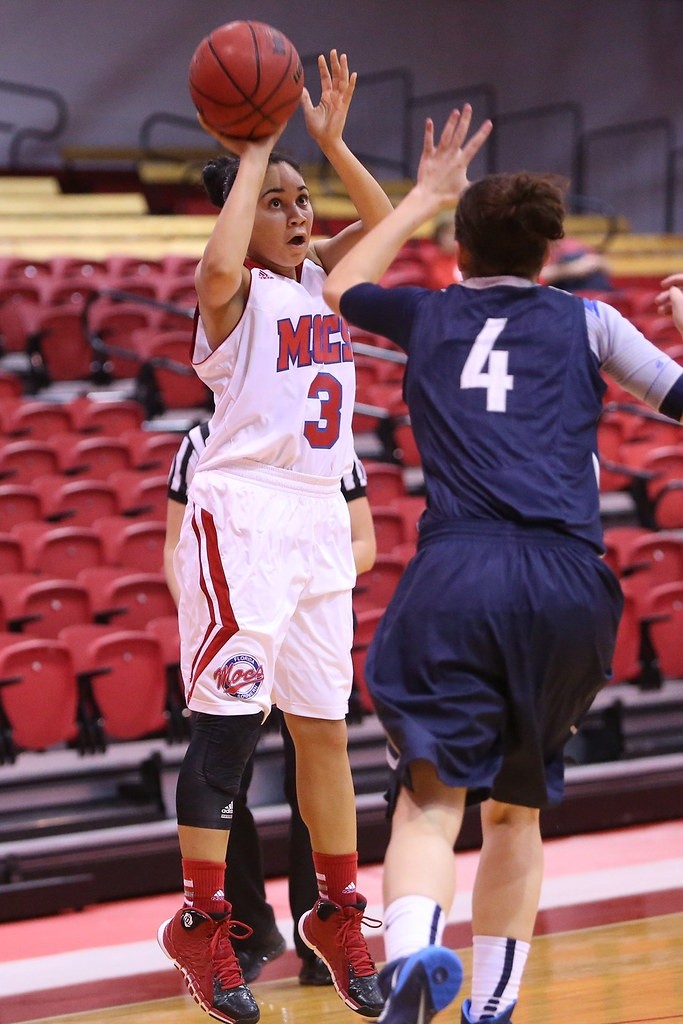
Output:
[298,948,334,986]
[229,921,284,986]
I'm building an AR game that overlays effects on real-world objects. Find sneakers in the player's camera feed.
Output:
[299,890,388,1018]
[455,995,517,1024]
[157,903,258,1024]
[374,943,463,1024]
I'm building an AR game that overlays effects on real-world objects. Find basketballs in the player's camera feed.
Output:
[188,20,305,139]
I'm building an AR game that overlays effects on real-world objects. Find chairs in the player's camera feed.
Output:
[0,153,683,843]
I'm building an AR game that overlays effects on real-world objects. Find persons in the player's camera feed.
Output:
[154,49,397,1024]
[164,417,376,987]
[321,102,683,1024]
